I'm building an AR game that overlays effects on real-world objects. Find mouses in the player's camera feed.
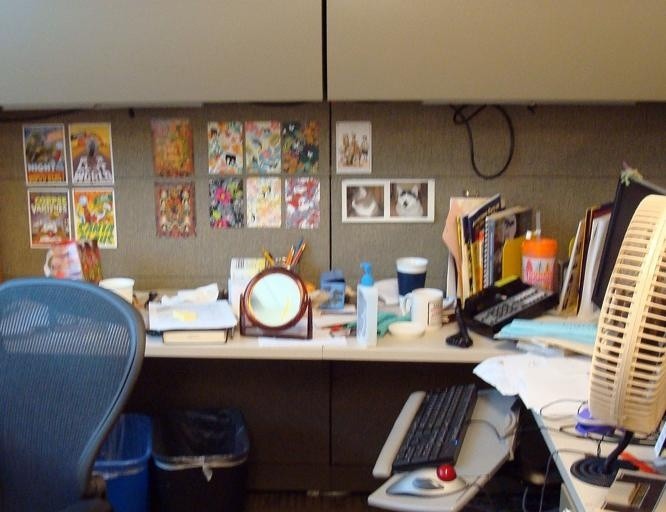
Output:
[386,463,466,496]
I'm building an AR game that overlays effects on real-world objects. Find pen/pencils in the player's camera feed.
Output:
[261,238,306,272]
[231,327,235,338]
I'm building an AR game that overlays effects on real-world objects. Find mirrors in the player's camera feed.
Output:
[237,265,314,341]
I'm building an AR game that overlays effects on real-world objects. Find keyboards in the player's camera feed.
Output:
[392,383,477,471]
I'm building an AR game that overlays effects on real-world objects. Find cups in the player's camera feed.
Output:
[395,255,430,307]
[401,287,445,331]
[98,278,135,305]
[521,238,558,292]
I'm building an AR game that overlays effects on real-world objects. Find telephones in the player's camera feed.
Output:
[460,275,559,339]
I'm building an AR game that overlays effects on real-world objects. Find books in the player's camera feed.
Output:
[313,304,356,330]
[160,297,232,346]
[444,192,621,360]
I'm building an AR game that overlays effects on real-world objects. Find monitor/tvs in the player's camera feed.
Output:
[591,173,666,309]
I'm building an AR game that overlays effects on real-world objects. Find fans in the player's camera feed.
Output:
[568,193,665,485]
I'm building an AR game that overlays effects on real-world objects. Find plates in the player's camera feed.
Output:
[388,321,426,336]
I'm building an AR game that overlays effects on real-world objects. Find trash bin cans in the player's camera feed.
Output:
[91,409,250,512]
